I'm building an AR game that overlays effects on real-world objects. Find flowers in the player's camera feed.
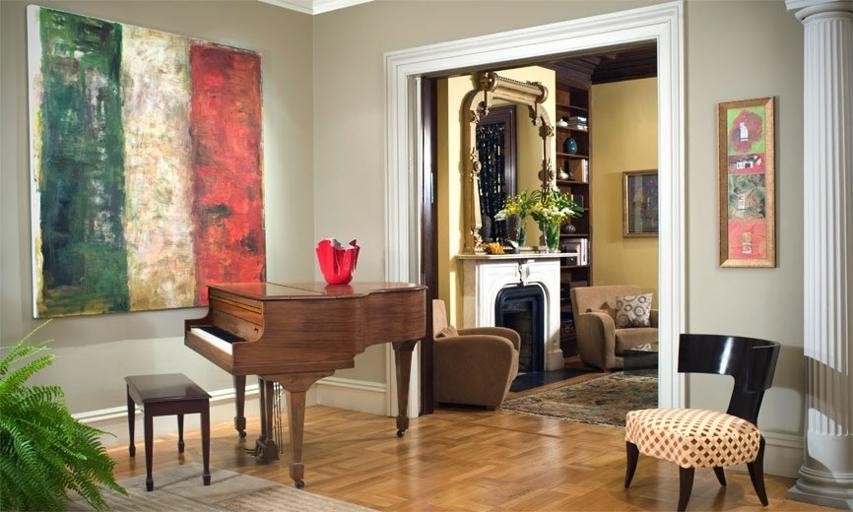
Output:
[532,188,584,249]
[493,187,541,245]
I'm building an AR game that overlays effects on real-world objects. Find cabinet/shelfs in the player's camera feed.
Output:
[557,74,593,359]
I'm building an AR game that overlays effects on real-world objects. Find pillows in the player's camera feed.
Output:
[615,293,653,329]
[439,324,458,337]
[586,307,609,315]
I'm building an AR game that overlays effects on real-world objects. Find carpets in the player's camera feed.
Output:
[66,463,380,512]
[501,370,658,429]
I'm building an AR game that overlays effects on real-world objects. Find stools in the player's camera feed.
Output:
[124,374,211,491]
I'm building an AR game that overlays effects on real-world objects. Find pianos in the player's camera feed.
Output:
[185,281,428,489]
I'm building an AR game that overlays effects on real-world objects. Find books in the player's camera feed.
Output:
[567,115,588,132]
[561,237,589,267]
[567,159,589,183]
[566,192,585,208]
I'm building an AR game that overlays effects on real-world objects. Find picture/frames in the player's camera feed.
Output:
[622,169,658,238]
[475,103,517,246]
[718,95,777,268]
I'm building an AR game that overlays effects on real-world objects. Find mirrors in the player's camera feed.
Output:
[464,73,554,254]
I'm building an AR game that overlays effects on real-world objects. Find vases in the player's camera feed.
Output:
[516,217,527,247]
[543,221,560,253]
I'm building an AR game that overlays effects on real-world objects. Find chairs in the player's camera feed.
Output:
[623,333,781,512]
[432,299,521,411]
[570,284,658,372]
